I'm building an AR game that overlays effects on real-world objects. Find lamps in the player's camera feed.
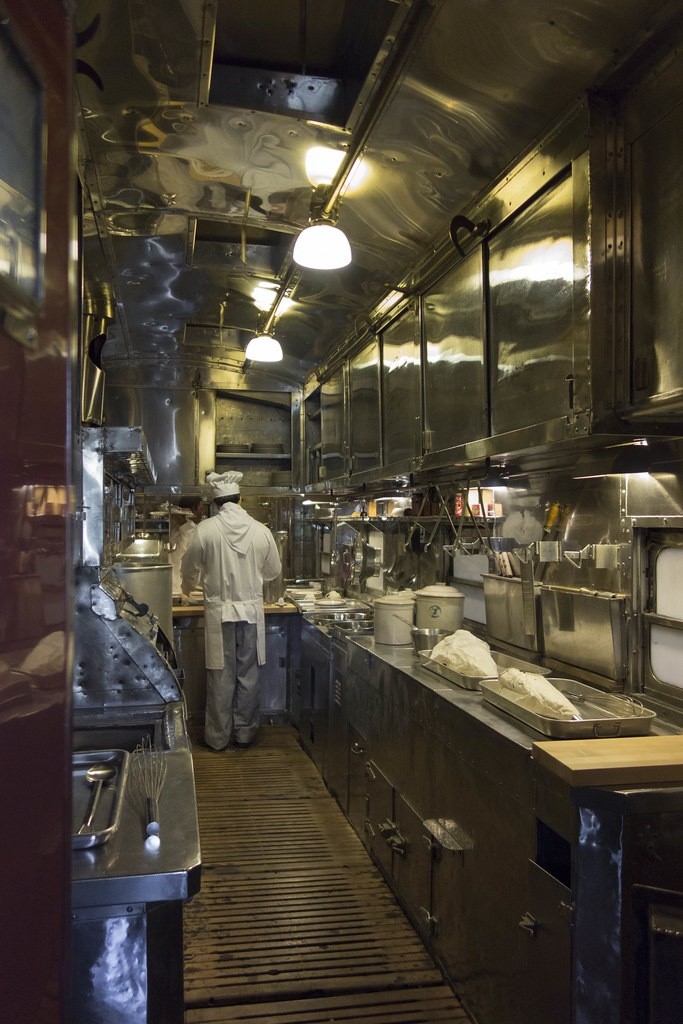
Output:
[245,324,283,362]
[293,205,351,270]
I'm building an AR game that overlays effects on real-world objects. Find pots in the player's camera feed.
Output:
[392,613,456,652]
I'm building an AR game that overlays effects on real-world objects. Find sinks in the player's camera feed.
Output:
[295,600,314,604]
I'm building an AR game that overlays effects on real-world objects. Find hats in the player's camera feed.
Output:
[206,470,243,499]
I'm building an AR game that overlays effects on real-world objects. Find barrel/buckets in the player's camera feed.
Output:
[415,583,464,633]
[395,589,420,600]
[373,595,415,645]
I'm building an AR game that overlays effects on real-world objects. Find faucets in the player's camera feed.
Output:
[295,578,327,595]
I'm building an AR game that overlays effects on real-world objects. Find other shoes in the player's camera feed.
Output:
[197,735,226,751]
[236,734,257,747]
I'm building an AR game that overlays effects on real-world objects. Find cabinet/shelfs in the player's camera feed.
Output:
[304,158,575,488]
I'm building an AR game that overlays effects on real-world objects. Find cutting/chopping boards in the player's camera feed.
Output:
[532,734,683,786]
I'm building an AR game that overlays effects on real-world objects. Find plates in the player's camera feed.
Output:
[314,599,346,606]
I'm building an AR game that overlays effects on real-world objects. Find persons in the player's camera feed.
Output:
[174,496,203,592]
[180,470,282,751]
